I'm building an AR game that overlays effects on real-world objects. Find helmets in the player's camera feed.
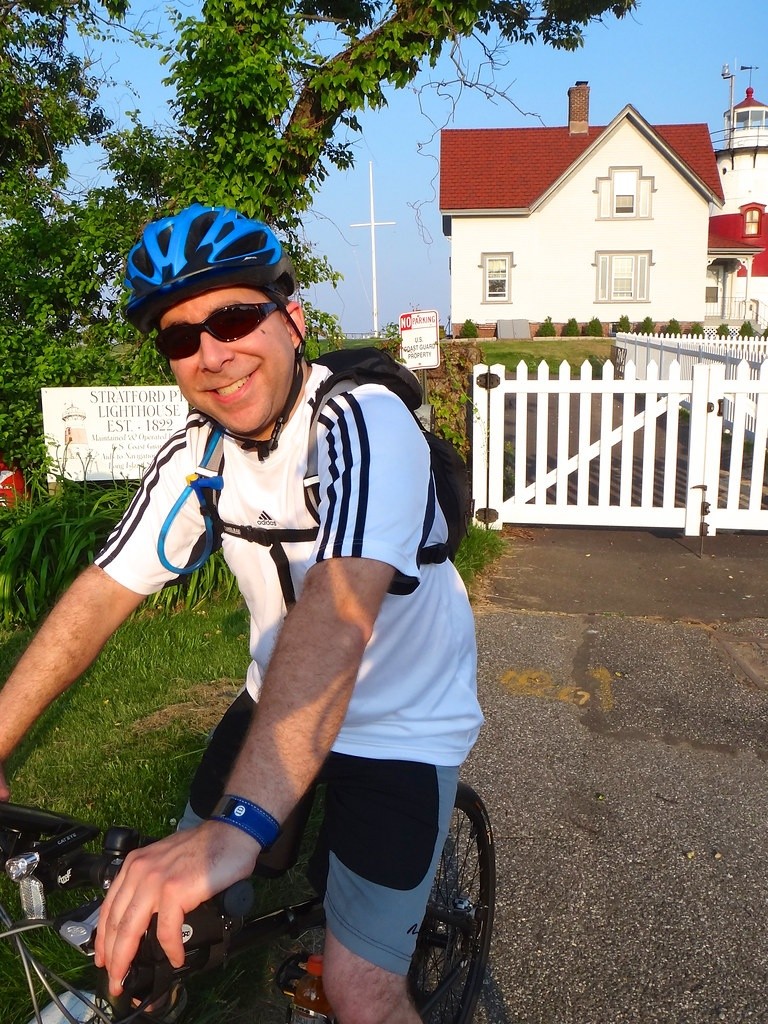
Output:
[120,203,283,334]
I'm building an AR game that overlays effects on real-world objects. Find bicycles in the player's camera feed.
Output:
[0,776,496,1024]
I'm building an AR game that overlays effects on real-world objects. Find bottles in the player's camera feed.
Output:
[290,954,338,1024]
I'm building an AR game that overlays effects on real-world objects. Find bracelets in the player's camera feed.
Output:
[209,795,280,850]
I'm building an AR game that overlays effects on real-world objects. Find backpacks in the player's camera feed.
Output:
[199,347,473,572]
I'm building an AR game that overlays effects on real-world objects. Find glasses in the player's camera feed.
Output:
[154,303,279,360]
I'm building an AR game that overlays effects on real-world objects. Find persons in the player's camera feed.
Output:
[0,202,484,1024]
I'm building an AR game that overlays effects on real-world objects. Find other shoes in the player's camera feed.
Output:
[30,979,186,1024]
